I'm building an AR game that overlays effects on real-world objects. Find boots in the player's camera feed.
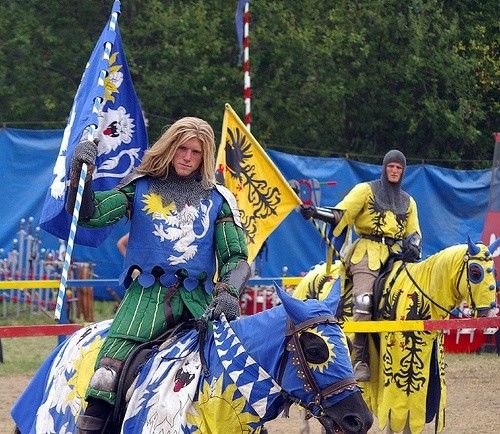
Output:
[75,415,104,434]
[349,313,371,381]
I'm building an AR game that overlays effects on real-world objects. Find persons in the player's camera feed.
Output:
[300,149,423,381]
[63,115,253,434]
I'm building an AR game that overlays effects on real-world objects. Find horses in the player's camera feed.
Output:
[291,233,500,434]
[11,276,374,433]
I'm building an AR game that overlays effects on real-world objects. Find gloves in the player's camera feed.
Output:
[401,233,421,262]
[65,137,101,222]
[204,259,251,322]
[300,205,335,225]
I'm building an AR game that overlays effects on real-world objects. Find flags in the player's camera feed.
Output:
[209,108,300,282]
[37,18,150,248]
